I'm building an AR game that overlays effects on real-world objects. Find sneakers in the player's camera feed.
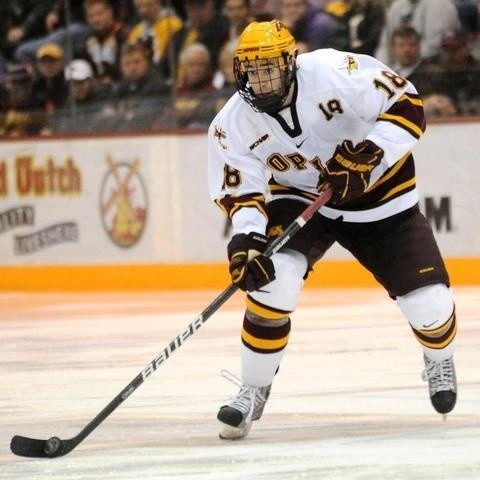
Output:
[422,354,457,413]
[218,369,270,426]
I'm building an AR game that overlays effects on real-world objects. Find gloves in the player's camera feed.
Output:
[316,140,383,206]
[228,232,275,291]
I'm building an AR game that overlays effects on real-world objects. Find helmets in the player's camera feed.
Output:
[233,19,298,112]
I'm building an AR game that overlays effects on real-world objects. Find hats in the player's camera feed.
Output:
[37,44,93,81]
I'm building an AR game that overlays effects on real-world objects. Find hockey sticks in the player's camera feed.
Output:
[11,186,335,458]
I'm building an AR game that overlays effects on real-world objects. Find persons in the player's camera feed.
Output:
[0,0,478,138]
[205,20,457,427]
